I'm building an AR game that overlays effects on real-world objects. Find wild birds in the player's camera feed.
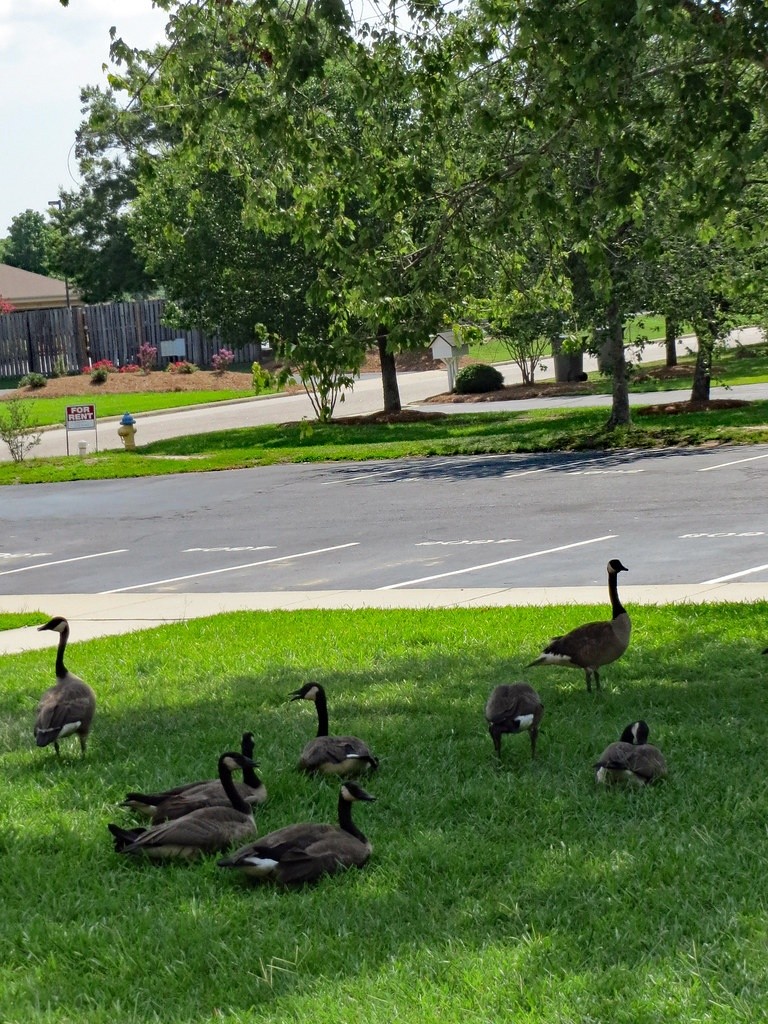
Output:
[592,719,672,790]
[485,683,544,761]
[523,559,632,691]
[108,732,267,867]
[288,681,380,782]
[217,781,380,888]
[33,616,95,759]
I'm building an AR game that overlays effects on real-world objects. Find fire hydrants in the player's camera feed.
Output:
[117,411,138,451]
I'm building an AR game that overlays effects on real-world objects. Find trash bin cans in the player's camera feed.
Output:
[594,326,627,371]
[552,335,587,382]
